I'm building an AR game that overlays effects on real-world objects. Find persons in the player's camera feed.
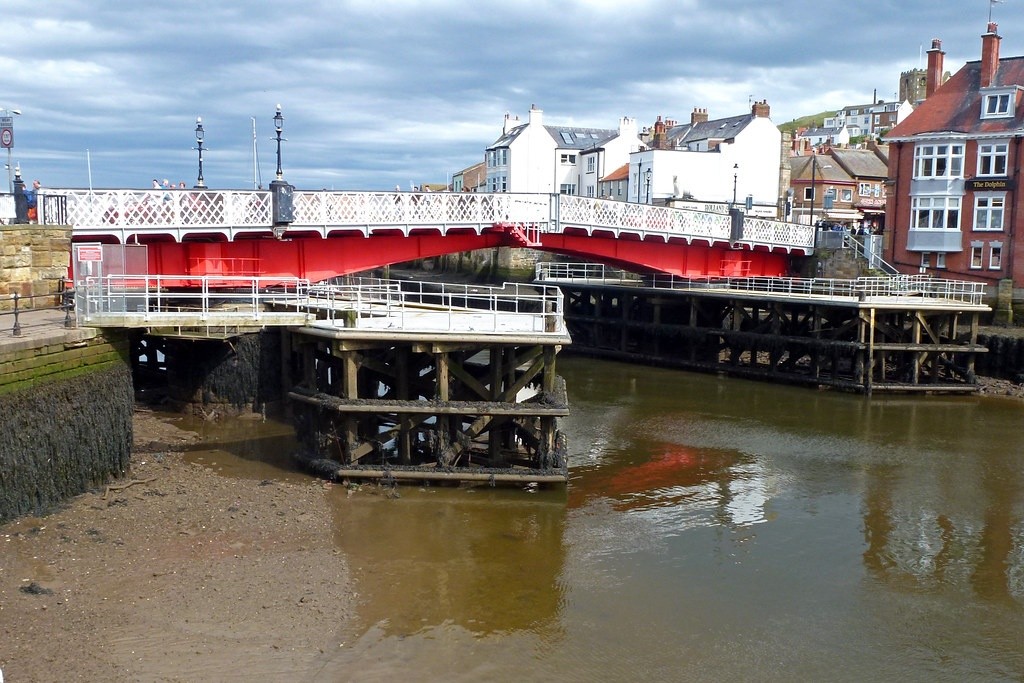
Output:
[815,220,881,236]
[23,178,188,225]
[394,184,476,219]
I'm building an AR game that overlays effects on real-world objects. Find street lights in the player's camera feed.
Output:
[644,167,652,205]
[0,108,22,193]
[733,163,740,206]
[267,104,287,182]
[191,116,210,188]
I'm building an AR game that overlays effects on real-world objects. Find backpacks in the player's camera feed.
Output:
[24,190,36,209]
